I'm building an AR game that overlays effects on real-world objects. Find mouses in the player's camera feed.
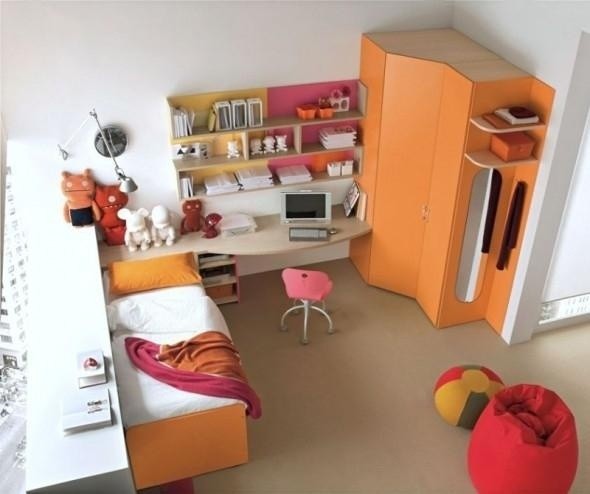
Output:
[329,226,337,235]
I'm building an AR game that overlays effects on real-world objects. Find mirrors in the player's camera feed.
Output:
[453,168,502,302]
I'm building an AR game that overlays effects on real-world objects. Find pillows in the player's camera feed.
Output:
[108,251,202,296]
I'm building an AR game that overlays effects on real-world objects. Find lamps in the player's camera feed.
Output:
[57,108,138,193]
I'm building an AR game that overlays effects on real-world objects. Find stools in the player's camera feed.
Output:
[280,268,335,345]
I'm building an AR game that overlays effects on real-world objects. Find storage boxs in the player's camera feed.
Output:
[220,212,258,239]
[206,285,232,299]
[489,132,537,162]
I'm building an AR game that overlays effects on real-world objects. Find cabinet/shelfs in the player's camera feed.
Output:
[164,79,368,203]
[348,34,555,336]
[194,253,240,304]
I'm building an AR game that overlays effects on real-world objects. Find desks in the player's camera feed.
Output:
[97,204,372,277]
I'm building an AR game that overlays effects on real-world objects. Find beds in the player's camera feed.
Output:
[103,270,248,490]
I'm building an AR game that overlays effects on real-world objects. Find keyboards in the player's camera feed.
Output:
[288,225,328,242]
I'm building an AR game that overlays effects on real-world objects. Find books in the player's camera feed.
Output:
[482,110,544,129]
[250,101,263,127]
[342,182,359,216]
[219,104,231,129]
[236,169,274,190]
[494,105,539,126]
[171,103,195,139]
[357,192,367,222]
[276,166,313,185]
[199,255,235,284]
[207,109,215,131]
[319,126,357,148]
[181,174,194,197]
[234,103,246,129]
[203,171,241,195]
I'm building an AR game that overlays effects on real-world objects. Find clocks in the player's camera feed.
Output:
[95,125,127,157]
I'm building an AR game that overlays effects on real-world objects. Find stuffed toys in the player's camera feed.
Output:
[263,137,275,154]
[227,140,238,158]
[275,135,287,150]
[62,169,101,226]
[92,180,129,246]
[148,205,175,248]
[116,207,151,252]
[181,201,219,238]
[250,139,263,157]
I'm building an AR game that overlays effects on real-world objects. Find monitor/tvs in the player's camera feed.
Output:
[281,192,332,225]
